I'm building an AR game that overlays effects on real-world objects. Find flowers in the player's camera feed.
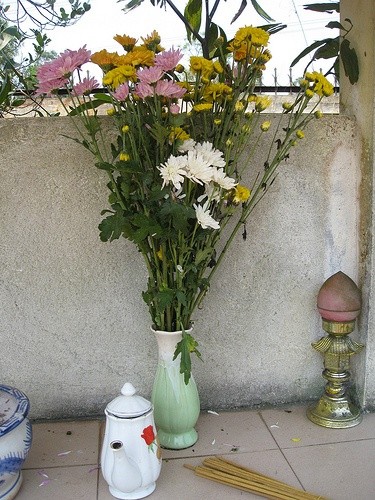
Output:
[27,15,335,385]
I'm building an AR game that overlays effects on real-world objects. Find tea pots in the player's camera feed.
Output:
[102,383,162,500]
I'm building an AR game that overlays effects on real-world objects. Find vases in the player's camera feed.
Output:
[147,322,205,449]
